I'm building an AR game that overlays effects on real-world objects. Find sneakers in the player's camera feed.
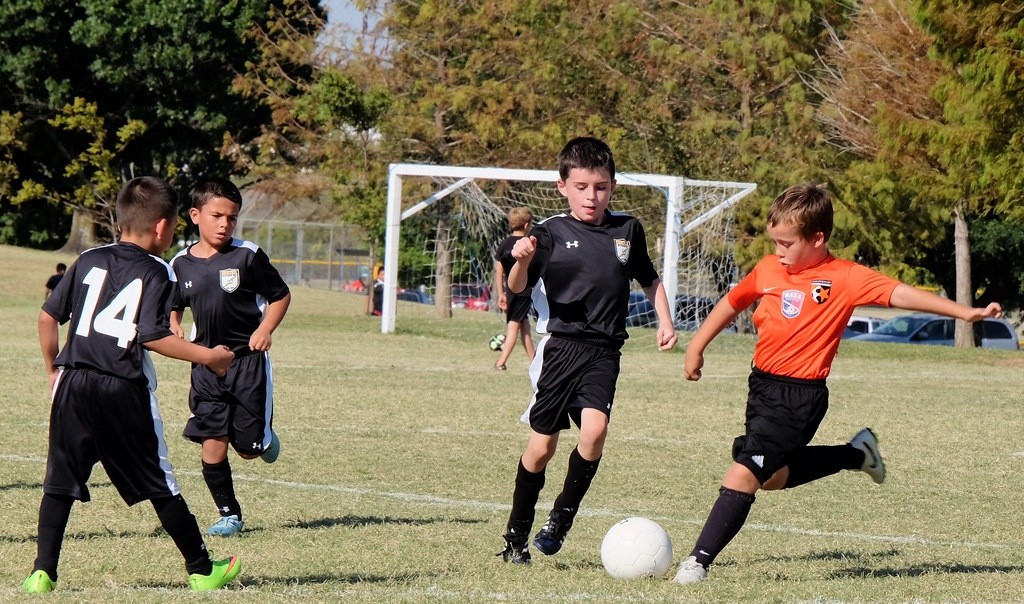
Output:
[495,523,531,564]
[22,570,58,594]
[188,550,241,591]
[532,507,577,555]
[208,515,245,535]
[849,427,886,484]
[672,555,706,585]
[260,430,280,463]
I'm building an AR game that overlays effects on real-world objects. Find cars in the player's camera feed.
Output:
[847,315,889,334]
[844,312,1021,352]
[450,283,491,312]
[396,289,435,305]
[624,289,737,334]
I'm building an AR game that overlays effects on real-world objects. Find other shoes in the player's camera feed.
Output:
[494,360,506,370]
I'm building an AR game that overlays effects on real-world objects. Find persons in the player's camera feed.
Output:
[343,277,366,292]
[45,263,67,301]
[672,186,1001,582]
[365,267,397,317]
[495,208,538,369]
[397,287,423,302]
[22,175,241,595]
[169,179,292,536]
[498,136,678,564]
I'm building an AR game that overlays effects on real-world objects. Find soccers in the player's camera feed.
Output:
[599,516,674,582]
